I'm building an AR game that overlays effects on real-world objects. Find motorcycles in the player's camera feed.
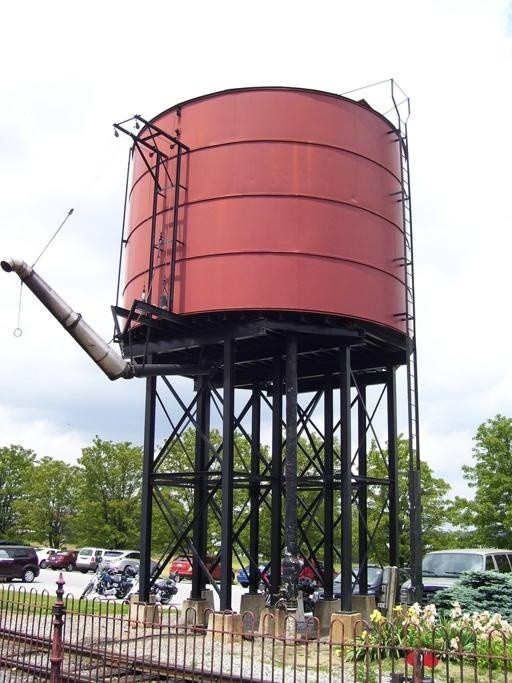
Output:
[76,555,179,607]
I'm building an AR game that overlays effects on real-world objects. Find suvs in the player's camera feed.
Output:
[398,546,512,622]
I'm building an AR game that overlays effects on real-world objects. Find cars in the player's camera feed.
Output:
[0,543,161,585]
[169,552,237,583]
[237,560,272,588]
[310,562,410,611]
